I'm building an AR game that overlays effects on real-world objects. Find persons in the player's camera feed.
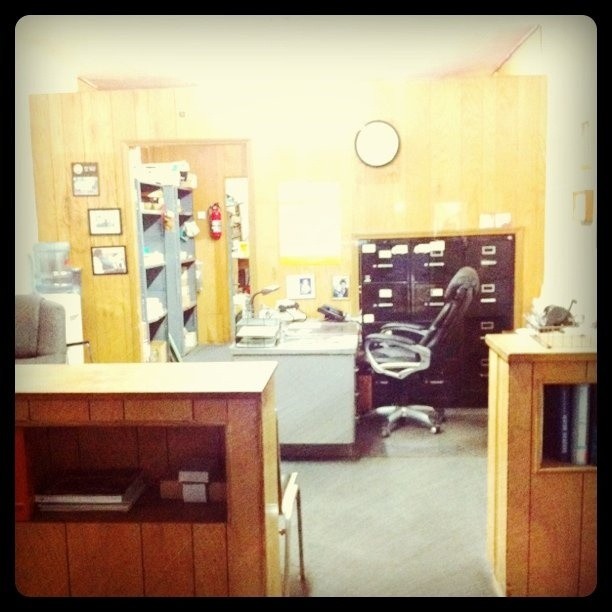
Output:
[338,280,348,296]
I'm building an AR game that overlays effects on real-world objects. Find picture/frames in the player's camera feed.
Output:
[90,245,128,275]
[88,207,122,235]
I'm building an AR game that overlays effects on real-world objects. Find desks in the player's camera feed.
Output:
[229,313,360,460]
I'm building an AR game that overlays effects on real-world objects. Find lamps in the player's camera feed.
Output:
[250,285,281,318]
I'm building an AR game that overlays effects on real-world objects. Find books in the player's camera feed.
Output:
[34,467,147,513]
[548,385,589,464]
[236,318,281,347]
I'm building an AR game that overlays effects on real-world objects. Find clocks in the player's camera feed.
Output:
[354,119,401,168]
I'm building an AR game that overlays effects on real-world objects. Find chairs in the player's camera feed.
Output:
[276,417,307,597]
[15,293,67,364]
[356,266,481,438]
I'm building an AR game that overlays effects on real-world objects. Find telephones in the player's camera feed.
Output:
[318,305,345,322]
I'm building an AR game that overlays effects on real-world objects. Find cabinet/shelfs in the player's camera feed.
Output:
[28,89,136,145]
[136,87,214,143]
[15,359,281,597]
[224,175,252,342]
[349,227,525,409]
[485,333,598,597]
[134,178,199,361]
